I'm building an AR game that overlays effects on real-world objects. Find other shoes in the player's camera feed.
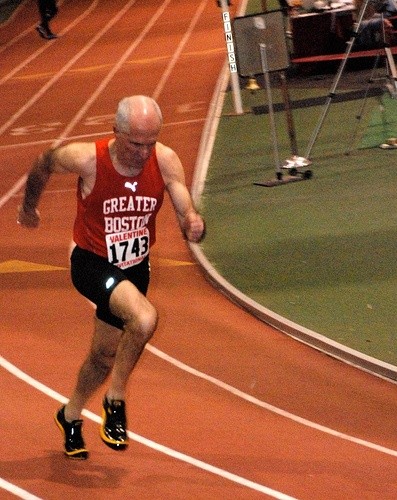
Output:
[34,22,57,40]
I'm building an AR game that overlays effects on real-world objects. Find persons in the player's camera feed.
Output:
[16,95,207,461]
[35,0,60,40]
[285,0,397,52]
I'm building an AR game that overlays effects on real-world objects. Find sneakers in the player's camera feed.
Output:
[100,393,130,451]
[52,404,88,459]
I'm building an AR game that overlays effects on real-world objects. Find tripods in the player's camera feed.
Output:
[344,0,397,156]
